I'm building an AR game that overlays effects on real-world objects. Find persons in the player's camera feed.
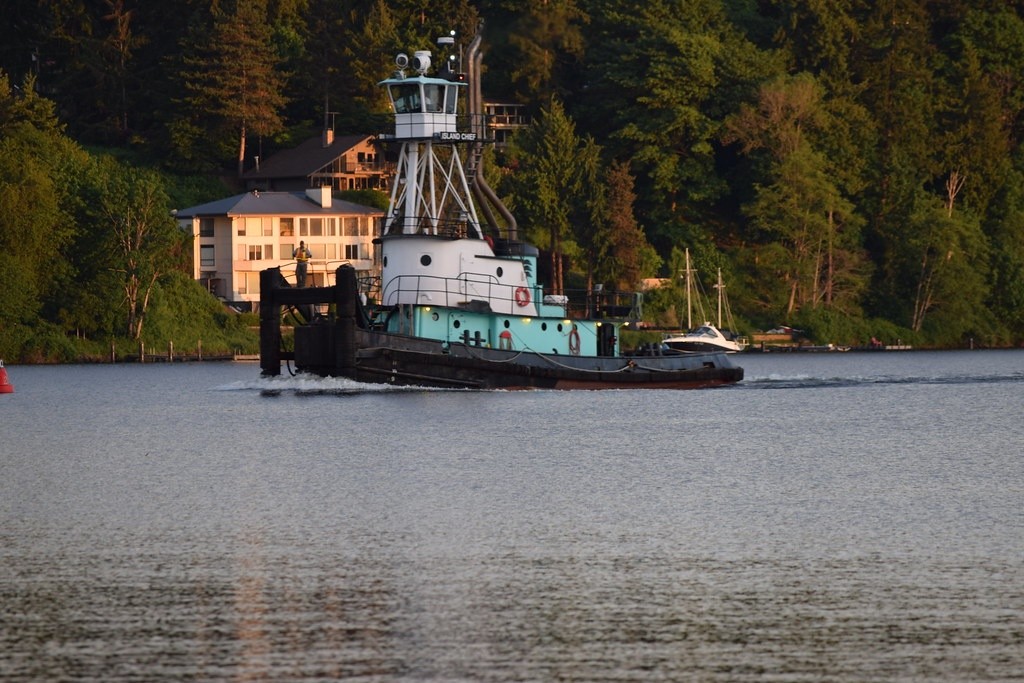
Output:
[291,240,312,288]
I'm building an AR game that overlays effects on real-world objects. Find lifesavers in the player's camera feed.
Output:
[484,235,494,250]
[515,286,531,307]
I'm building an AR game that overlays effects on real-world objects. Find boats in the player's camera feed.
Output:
[258,30,745,387]
[661,247,744,353]
